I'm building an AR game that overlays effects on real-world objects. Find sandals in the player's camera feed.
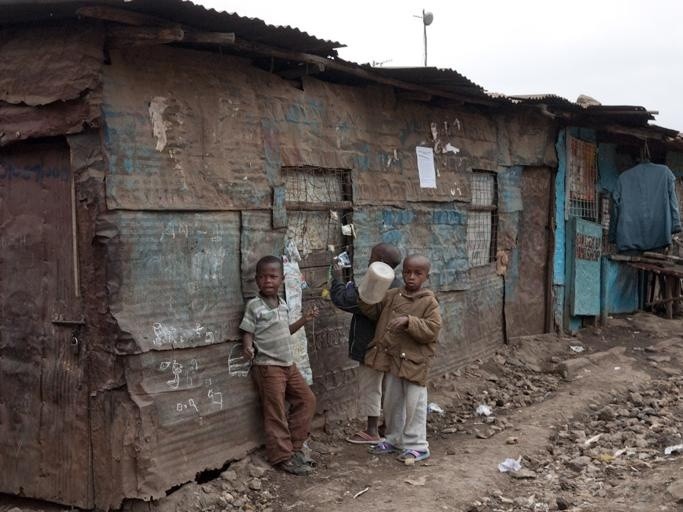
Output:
[397,448,430,462]
[368,442,405,454]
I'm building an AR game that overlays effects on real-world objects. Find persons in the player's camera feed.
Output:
[329,244,403,445]
[238,255,319,475]
[357,255,441,464]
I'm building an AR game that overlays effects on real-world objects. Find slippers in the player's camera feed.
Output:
[345,431,386,444]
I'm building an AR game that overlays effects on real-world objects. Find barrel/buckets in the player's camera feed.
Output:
[358,260,395,306]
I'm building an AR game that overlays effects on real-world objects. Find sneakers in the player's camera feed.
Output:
[280,462,313,476]
[295,450,317,464]
